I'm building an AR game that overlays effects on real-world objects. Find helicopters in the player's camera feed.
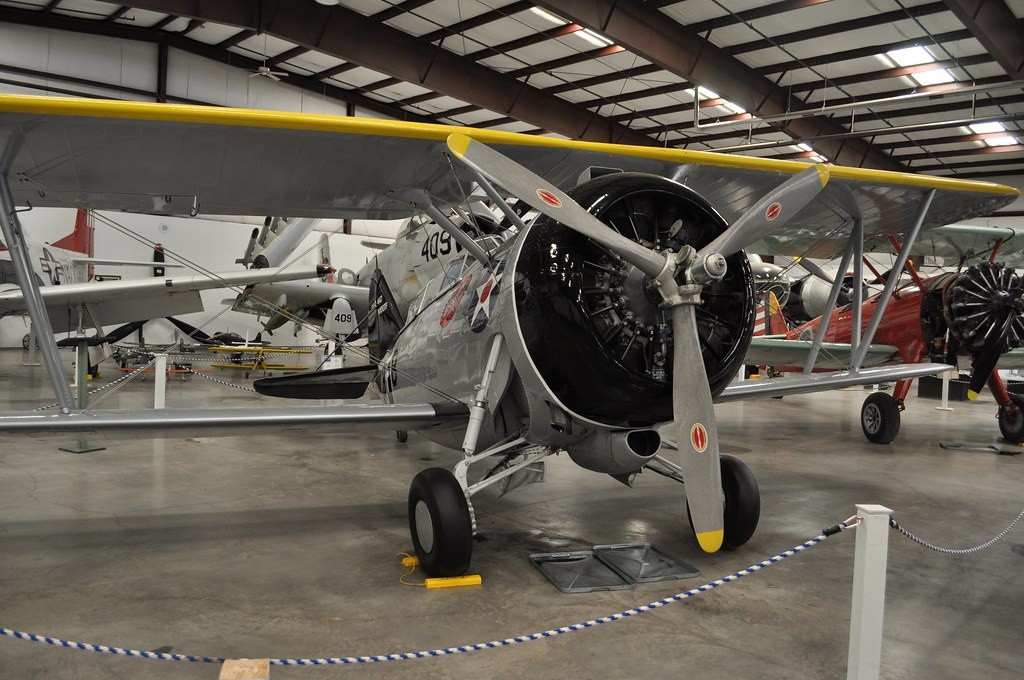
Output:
[1,91,1019,577]
